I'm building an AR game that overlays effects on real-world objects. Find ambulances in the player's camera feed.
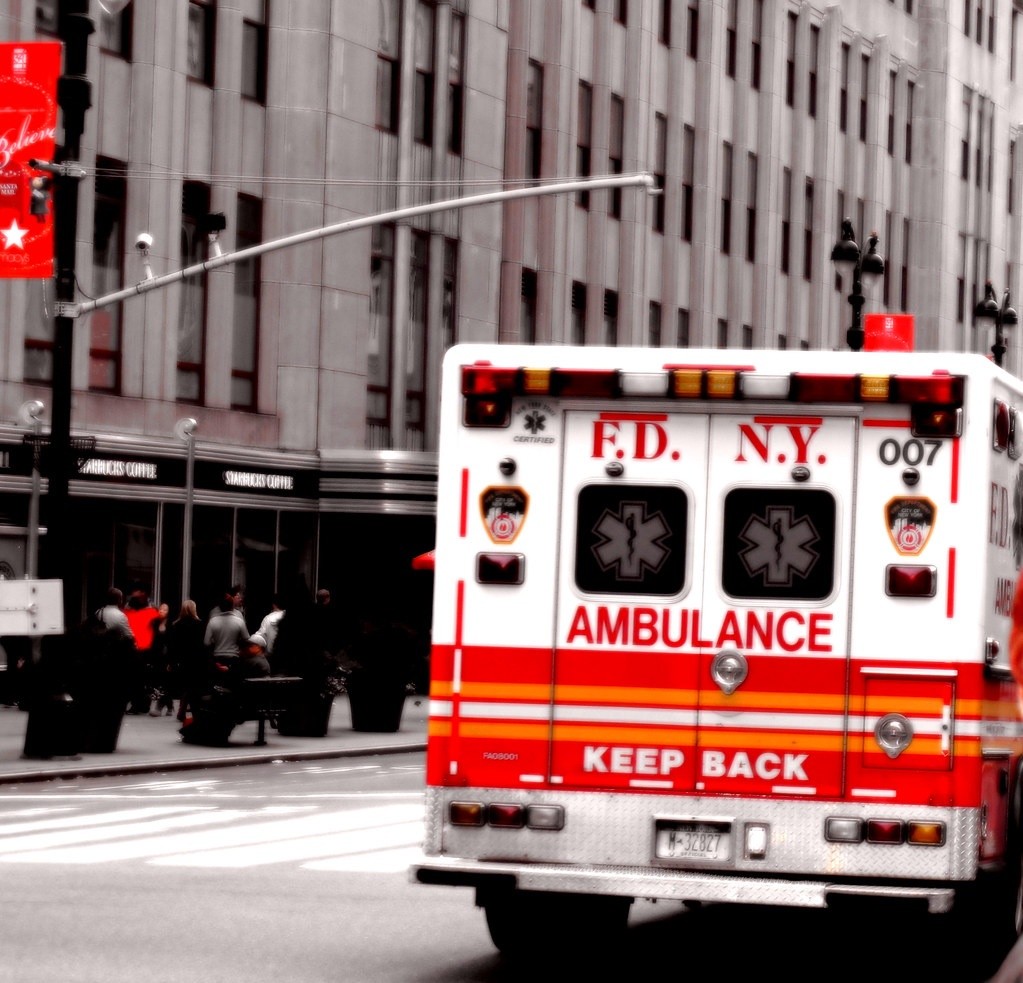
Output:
[406,310,1023,983]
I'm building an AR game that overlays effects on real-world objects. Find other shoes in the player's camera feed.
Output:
[150,710,162,717]
[166,708,173,716]
[127,707,141,715]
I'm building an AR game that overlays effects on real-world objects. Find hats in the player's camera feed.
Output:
[244,634,267,650]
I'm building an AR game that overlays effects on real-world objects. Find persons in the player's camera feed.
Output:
[94,585,330,748]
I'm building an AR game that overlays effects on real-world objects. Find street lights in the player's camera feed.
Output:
[829,215,887,352]
[974,280,1020,366]
